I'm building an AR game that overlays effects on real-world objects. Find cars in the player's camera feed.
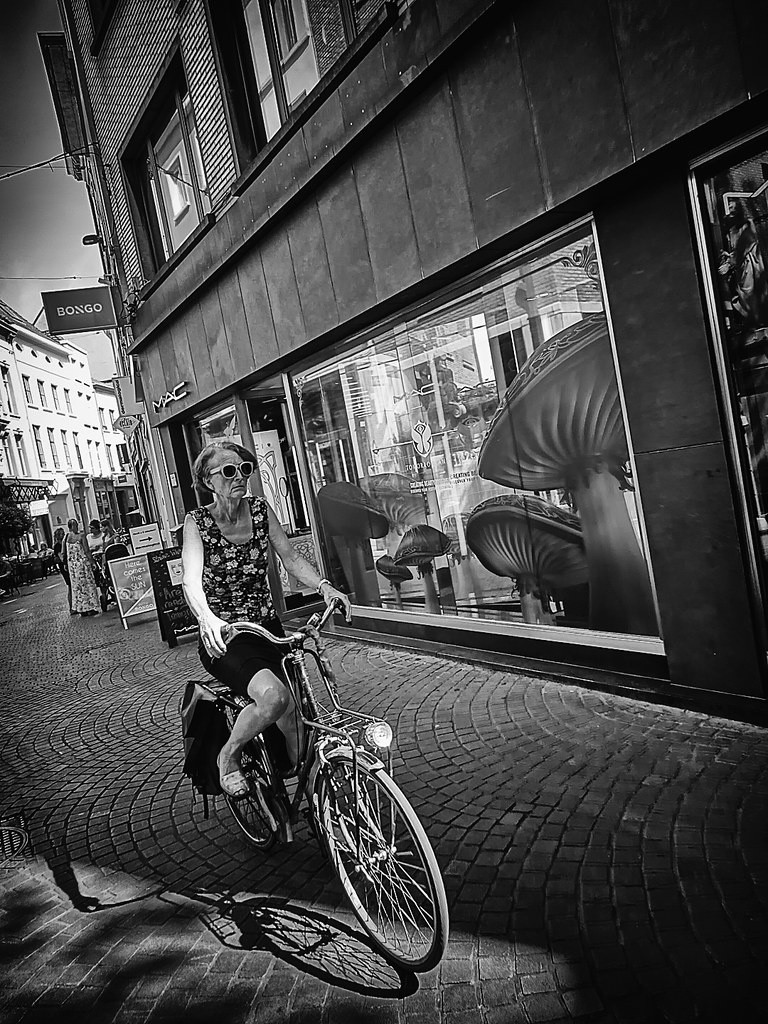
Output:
[206,597,450,975]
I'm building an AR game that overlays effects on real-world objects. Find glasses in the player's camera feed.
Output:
[88,526,96,529]
[209,459,253,481]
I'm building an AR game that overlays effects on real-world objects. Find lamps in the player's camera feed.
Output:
[82,234,99,245]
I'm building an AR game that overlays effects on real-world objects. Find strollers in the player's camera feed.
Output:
[93,543,132,613]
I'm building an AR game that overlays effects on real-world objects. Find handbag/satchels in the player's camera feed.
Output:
[62,554,67,565]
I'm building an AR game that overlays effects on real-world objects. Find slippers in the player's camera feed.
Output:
[216,752,249,797]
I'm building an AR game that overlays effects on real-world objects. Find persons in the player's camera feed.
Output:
[61,519,102,616]
[29,544,60,573]
[86,520,120,557]
[53,528,79,615]
[182,442,352,849]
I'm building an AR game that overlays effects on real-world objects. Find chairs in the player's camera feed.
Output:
[0,556,59,599]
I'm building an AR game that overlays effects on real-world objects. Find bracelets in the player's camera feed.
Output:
[316,579,332,596]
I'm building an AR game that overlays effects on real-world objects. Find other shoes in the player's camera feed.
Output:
[88,610,99,615]
[81,611,87,616]
